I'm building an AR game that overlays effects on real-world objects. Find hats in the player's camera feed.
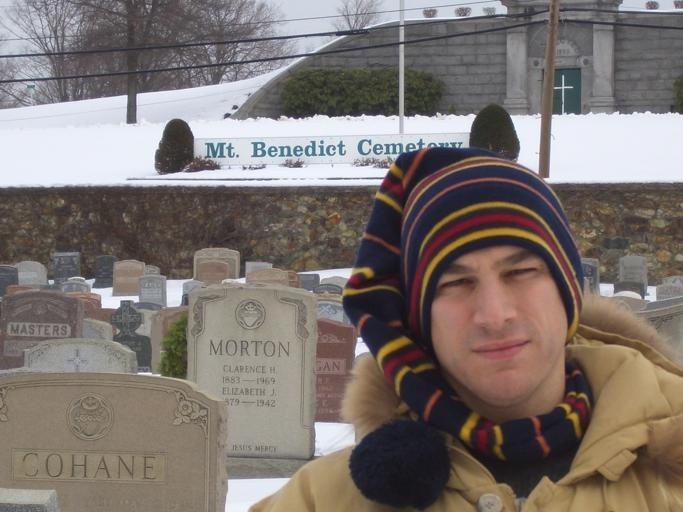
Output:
[399,155,584,346]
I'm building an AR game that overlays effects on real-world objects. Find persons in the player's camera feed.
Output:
[244,146,683,512]
